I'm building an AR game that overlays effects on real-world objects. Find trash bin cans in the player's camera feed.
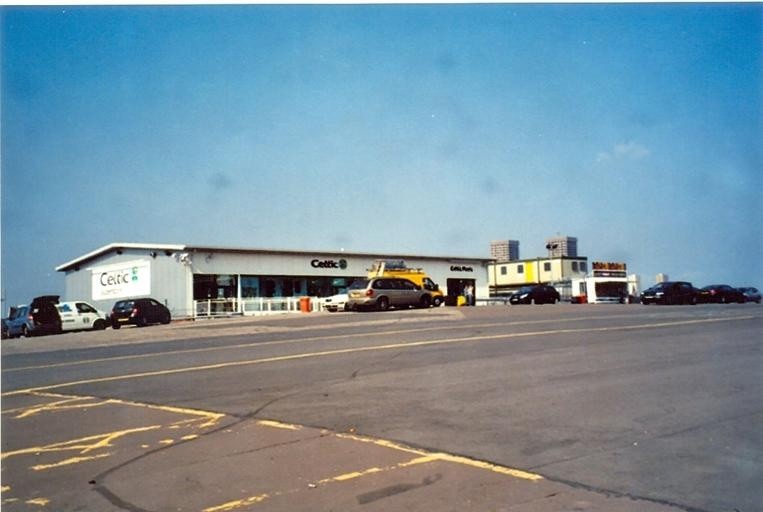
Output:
[300,297,310,312]
[579,295,586,303]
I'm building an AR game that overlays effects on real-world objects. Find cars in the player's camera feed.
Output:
[640,281,761,306]
[509,285,559,305]
[0,295,171,340]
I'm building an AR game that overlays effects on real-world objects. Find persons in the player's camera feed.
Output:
[467,281,473,306]
[462,283,468,306]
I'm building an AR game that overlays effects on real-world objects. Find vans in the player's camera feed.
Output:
[320,259,445,315]
